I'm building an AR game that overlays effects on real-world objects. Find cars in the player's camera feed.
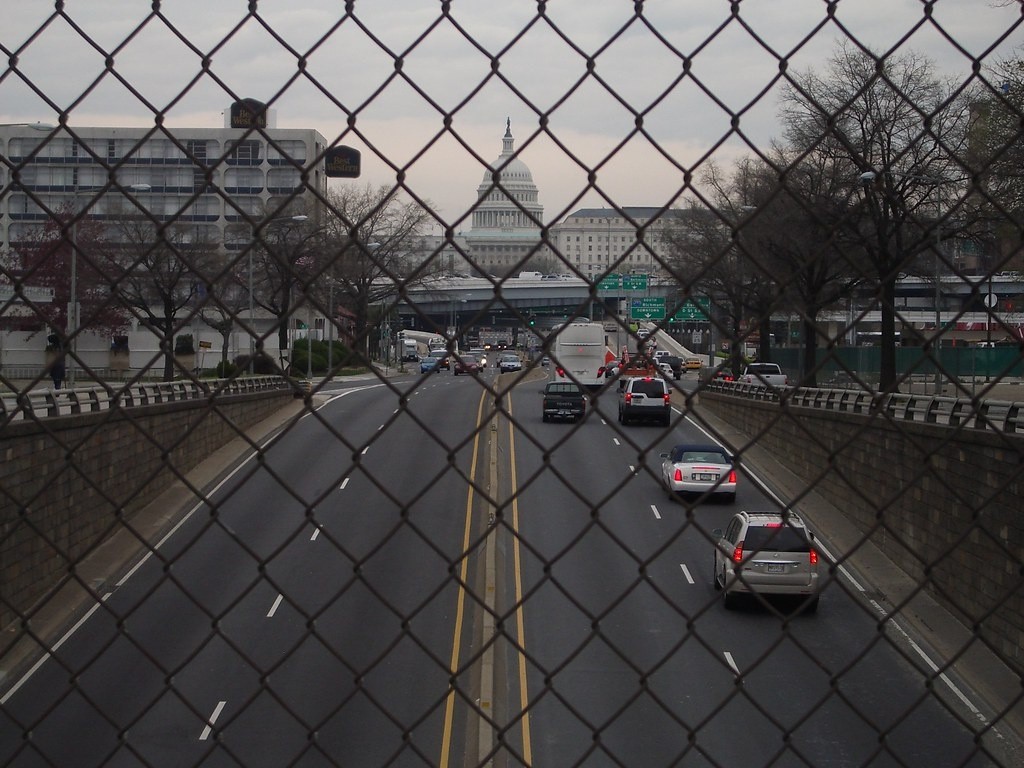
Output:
[419,357,441,374]
[499,355,523,374]
[711,366,734,387]
[445,268,659,282]
[396,330,508,372]
[659,443,737,504]
[452,355,480,376]
[602,315,687,386]
[683,356,703,369]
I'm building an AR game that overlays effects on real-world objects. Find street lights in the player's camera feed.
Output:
[742,203,792,350]
[328,241,381,380]
[67,183,152,387]
[250,215,308,378]
[859,169,941,397]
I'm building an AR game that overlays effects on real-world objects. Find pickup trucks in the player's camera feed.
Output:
[738,362,789,397]
[538,381,589,424]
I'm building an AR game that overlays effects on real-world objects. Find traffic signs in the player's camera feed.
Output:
[622,273,647,289]
[593,273,619,291]
[675,293,710,321]
[631,296,666,320]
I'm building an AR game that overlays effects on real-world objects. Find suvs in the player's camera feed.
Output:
[712,510,819,613]
[427,349,452,370]
[617,376,672,427]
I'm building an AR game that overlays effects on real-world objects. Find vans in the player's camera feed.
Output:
[548,322,609,389]
